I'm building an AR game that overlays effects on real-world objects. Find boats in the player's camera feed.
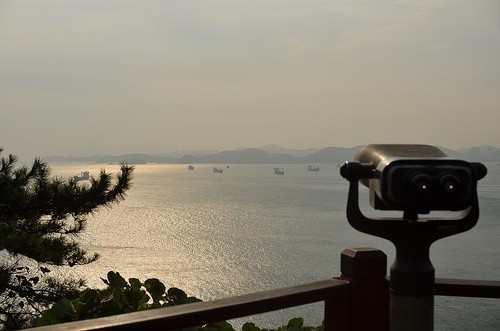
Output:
[226,165,229,168]
[188,165,194,170]
[213,168,224,173]
[273,168,284,175]
[307,165,320,171]
[73,171,89,181]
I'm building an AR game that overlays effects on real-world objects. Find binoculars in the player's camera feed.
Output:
[342,143,489,331]
